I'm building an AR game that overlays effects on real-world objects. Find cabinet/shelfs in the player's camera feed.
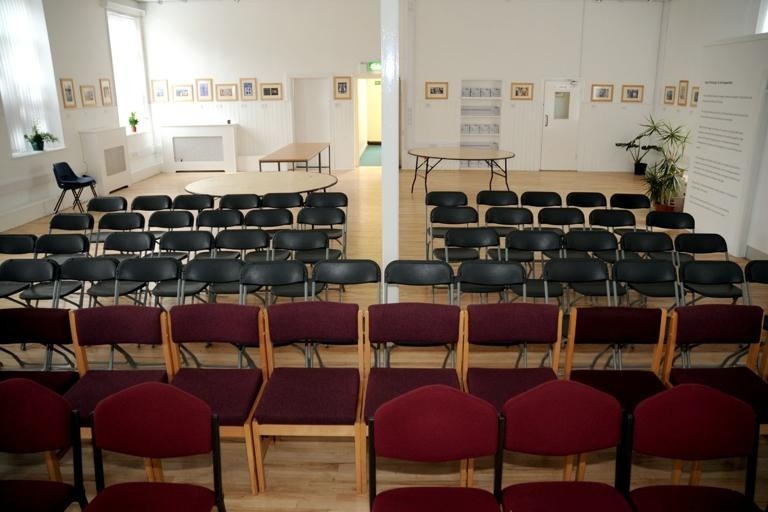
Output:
[160,121,239,173]
[79,126,133,196]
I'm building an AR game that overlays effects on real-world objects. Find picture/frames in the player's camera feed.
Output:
[60,76,116,108]
[332,76,352,100]
[149,78,282,104]
[511,82,534,100]
[591,83,614,101]
[621,84,644,103]
[664,80,699,106]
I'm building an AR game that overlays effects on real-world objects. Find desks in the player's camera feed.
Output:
[259,140,331,177]
[407,146,515,195]
[186,170,338,197]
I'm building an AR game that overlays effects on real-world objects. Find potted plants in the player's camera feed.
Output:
[128,112,139,133]
[23,124,59,151]
[615,135,663,176]
[639,113,694,211]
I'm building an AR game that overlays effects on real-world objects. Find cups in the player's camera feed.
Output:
[227,120,230,124]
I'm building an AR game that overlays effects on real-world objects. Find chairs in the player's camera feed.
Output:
[50,162,97,214]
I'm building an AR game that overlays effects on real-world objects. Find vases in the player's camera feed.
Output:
[425,81,448,99]
